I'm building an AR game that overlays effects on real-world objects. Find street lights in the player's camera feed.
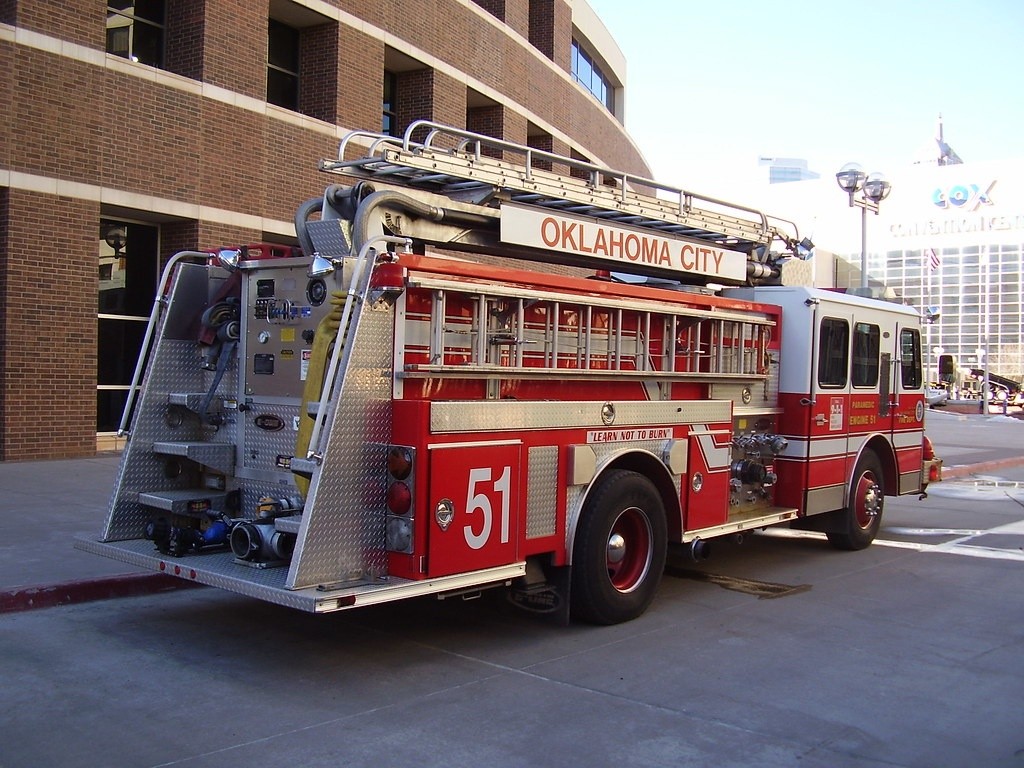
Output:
[932,346,944,387]
[835,163,892,288]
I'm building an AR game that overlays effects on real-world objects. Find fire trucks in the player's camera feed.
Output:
[72,121,958,631]
